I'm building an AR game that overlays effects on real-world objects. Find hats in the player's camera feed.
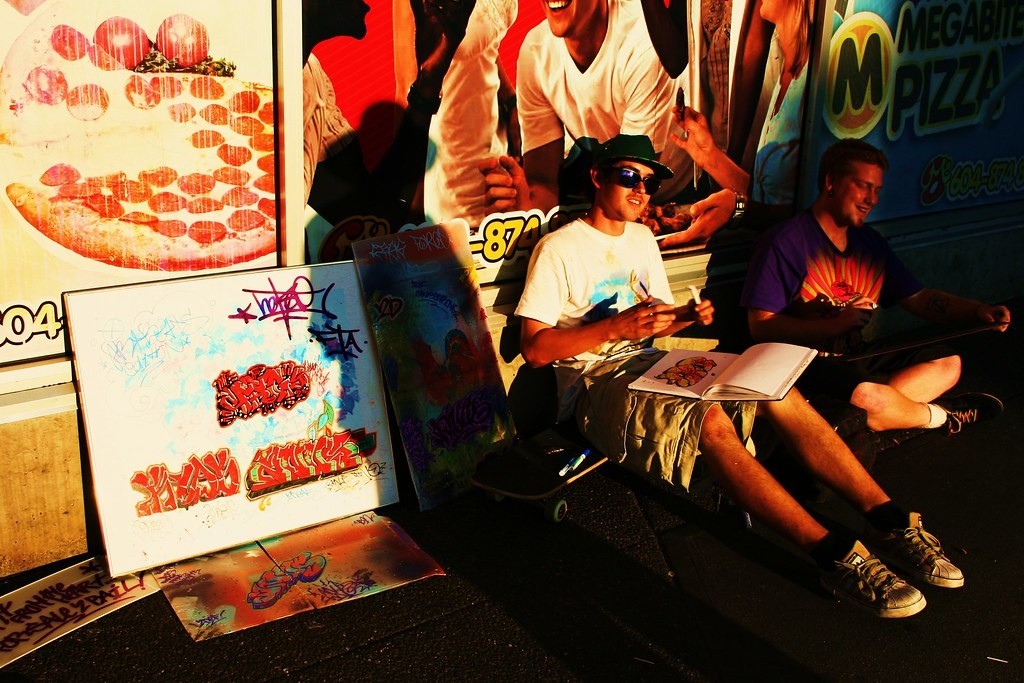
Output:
[587,134,675,180]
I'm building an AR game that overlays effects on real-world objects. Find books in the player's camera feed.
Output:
[627,343,818,401]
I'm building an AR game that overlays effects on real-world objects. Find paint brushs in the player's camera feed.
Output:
[638,281,651,298]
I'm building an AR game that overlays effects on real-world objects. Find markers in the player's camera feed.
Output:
[688,285,702,305]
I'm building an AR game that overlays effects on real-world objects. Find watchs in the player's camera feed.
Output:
[733,194,745,219]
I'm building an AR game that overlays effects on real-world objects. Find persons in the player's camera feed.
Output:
[746,139,1009,435]
[479,0,827,247]
[512,135,964,619]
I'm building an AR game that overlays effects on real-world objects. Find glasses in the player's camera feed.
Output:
[601,166,661,195]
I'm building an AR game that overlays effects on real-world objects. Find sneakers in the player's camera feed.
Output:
[880,511,965,588]
[834,408,927,452]
[818,541,927,617]
[928,389,1005,438]
